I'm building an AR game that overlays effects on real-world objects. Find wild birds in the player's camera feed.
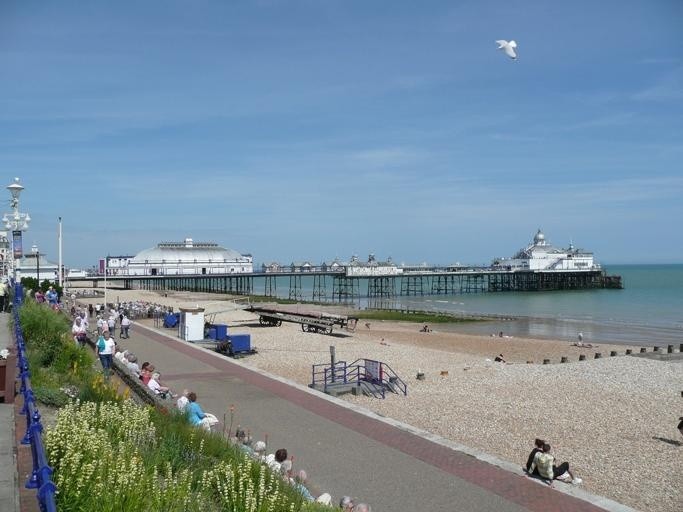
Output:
[494,38,519,60]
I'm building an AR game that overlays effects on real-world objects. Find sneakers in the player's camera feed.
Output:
[571,478,582,484]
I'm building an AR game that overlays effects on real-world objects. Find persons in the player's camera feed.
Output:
[493,352,506,362]
[523,443,583,489]
[526,437,558,479]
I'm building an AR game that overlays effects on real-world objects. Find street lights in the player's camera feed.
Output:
[3,197,31,306]
